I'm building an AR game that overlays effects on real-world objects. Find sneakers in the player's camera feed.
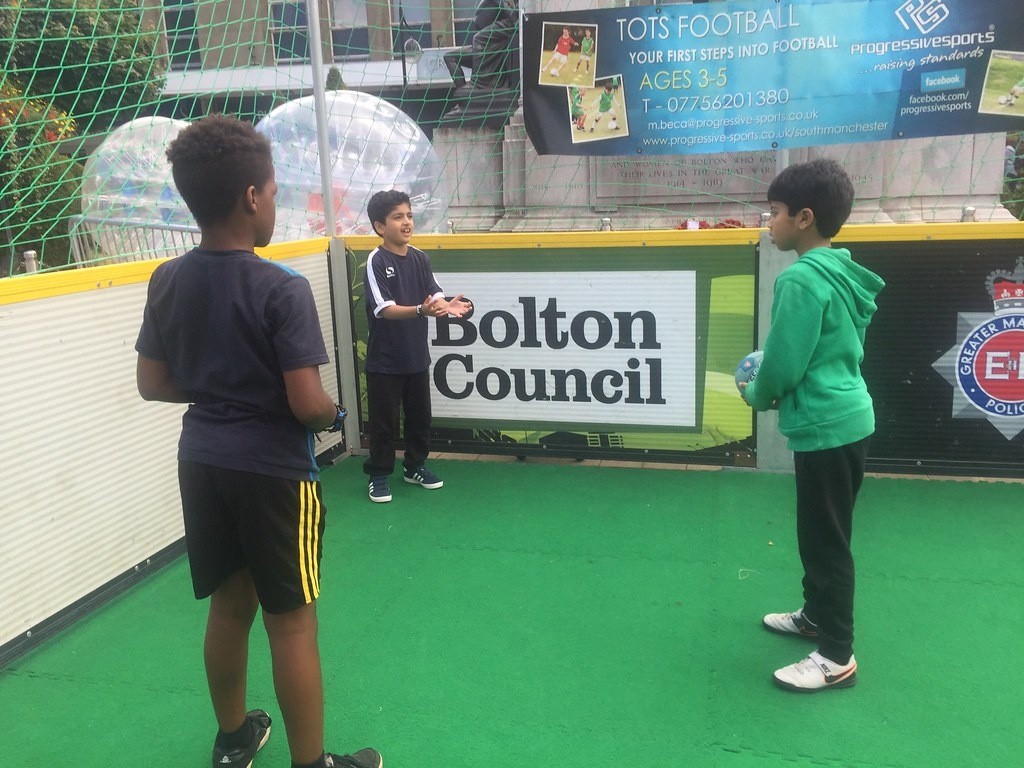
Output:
[404,466,443,489]
[324,748,383,768]
[212,709,272,768]
[368,475,392,502]
[773,649,858,692]
[763,608,819,639]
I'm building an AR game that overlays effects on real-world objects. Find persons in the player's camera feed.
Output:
[442,0,503,116]
[542,27,579,78]
[135,111,385,768]
[1007,75,1024,106]
[590,83,622,133]
[568,84,584,124]
[362,190,474,503]
[611,76,621,96]
[463,1,518,89]
[574,28,595,75]
[737,158,880,695]
[571,87,589,132]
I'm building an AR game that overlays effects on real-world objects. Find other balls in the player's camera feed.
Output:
[735,349,765,398]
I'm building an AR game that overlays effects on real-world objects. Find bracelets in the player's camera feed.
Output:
[314,403,348,442]
[416,304,428,319]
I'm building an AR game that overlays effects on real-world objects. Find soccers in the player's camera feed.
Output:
[550,68,558,77]
[608,121,616,129]
[999,96,1007,104]
